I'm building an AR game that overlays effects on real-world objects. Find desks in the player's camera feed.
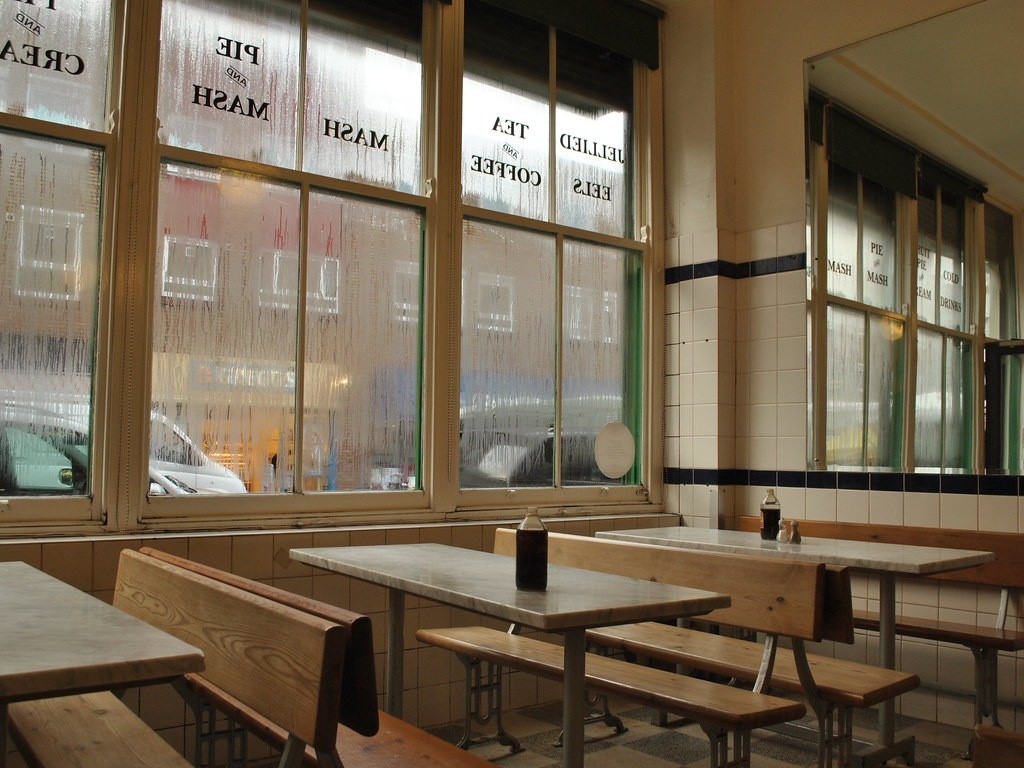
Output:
[1,562,205,698]
[288,544,734,764]
[597,525,996,768]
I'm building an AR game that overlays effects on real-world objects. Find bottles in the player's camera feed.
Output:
[516,507,548,591]
[789,521,801,544]
[776,519,788,543]
[760,489,780,540]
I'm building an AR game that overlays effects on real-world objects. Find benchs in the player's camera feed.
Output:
[6,515,1024,768]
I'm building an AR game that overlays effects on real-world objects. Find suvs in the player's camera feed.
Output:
[0,389,250,495]
